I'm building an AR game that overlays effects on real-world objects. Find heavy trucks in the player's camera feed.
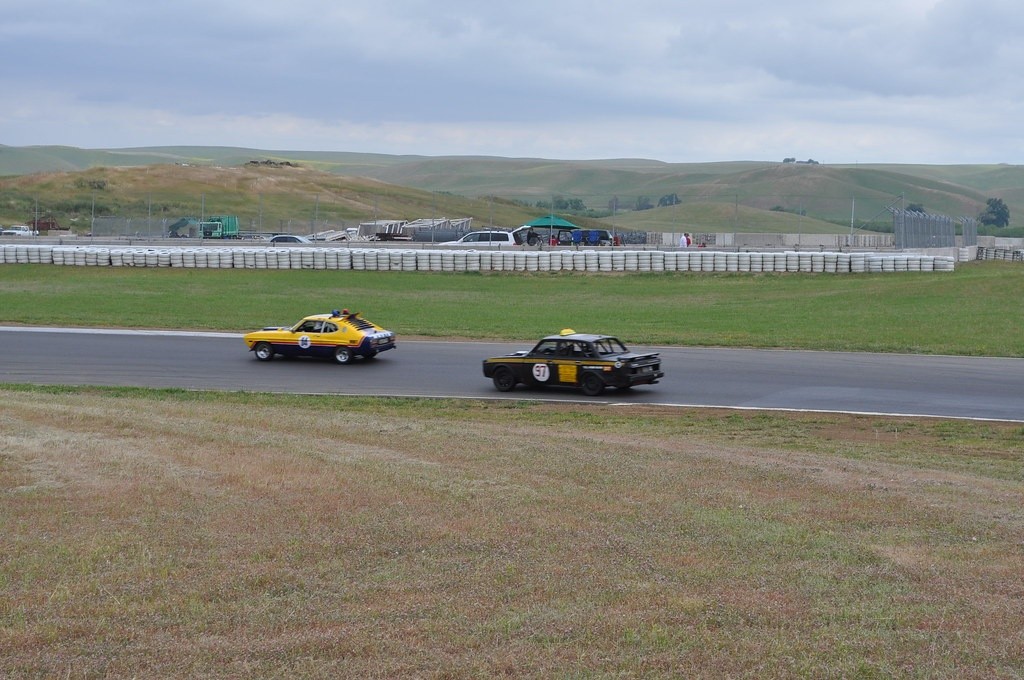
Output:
[198,216,239,239]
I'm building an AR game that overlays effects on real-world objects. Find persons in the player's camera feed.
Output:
[560,343,567,355]
[680,233,691,247]
[698,242,707,247]
[527,227,542,247]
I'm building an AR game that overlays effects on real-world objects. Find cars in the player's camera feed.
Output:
[557,229,613,247]
[483,329,664,397]
[242,309,396,365]
[438,225,531,246]
[268,235,312,243]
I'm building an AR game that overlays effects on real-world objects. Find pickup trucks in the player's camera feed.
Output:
[1,226,39,236]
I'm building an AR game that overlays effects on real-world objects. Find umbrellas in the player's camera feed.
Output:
[522,216,581,246]
